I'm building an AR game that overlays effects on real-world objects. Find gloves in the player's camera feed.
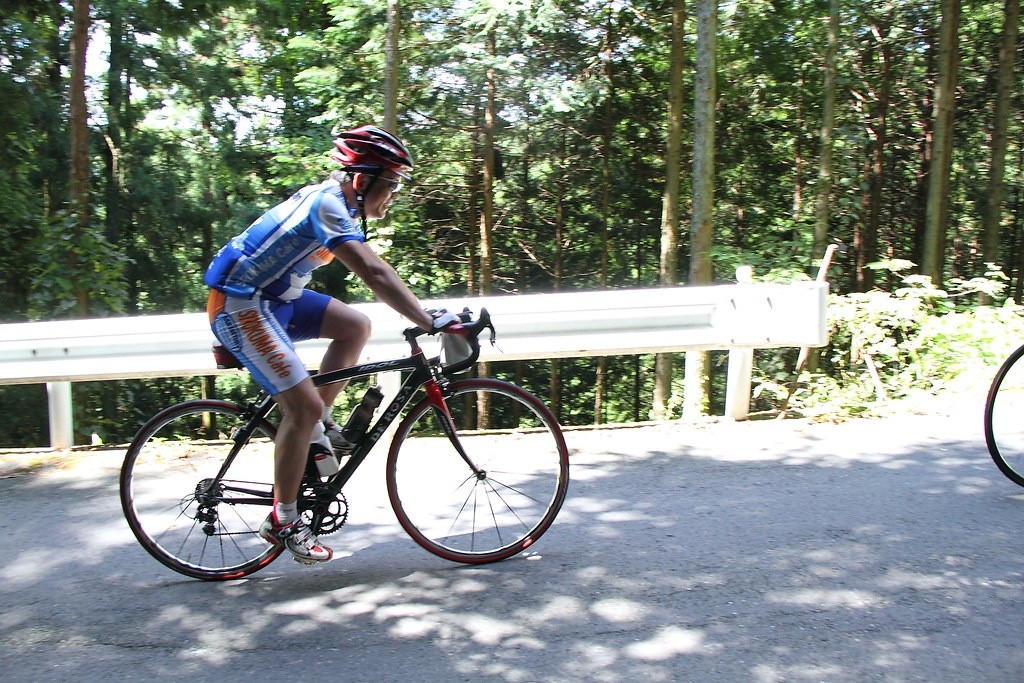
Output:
[431,309,461,333]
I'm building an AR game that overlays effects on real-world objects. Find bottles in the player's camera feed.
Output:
[340,384,384,444]
[308,419,340,477]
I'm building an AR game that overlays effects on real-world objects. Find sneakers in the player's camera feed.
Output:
[259,512,333,562]
[322,417,357,455]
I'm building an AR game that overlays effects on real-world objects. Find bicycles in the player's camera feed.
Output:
[120,307,570,581]
[983,344,1024,487]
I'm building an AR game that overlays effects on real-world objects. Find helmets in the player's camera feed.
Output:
[329,125,415,182]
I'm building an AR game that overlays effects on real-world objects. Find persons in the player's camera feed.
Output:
[204,125,462,562]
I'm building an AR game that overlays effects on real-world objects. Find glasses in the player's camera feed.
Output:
[347,170,404,194]
